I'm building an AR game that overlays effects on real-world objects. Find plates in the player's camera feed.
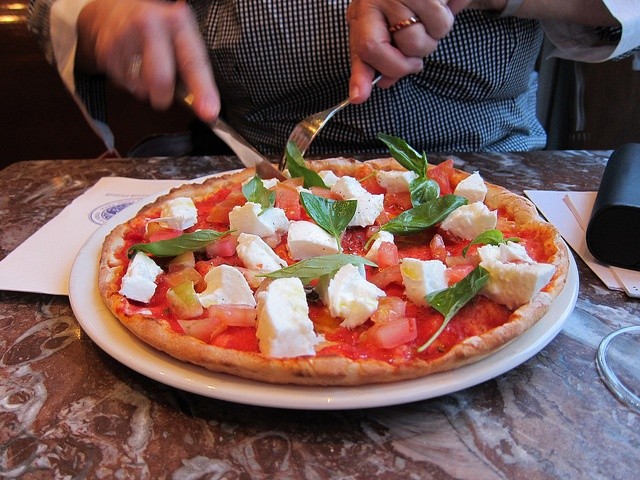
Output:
[67,158,581,410]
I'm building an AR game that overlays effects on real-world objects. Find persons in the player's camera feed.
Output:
[23,0,640,155]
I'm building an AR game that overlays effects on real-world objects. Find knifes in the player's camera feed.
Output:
[170,83,275,170]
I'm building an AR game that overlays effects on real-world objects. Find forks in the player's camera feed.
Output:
[278,73,385,171]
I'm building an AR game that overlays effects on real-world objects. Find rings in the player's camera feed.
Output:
[389,15,422,33]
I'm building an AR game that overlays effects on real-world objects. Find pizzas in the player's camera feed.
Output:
[96,157,570,383]
